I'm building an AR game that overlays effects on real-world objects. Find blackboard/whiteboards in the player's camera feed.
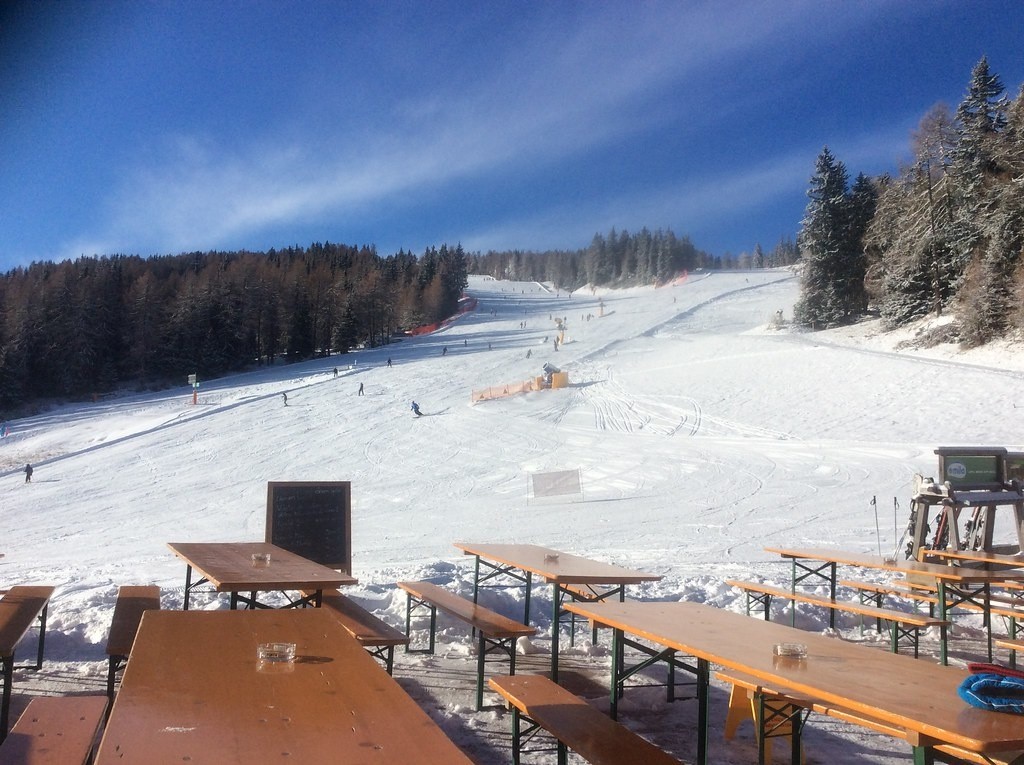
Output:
[265,481,352,570]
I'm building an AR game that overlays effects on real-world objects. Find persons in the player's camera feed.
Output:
[24,464,33,483]
[442,277,592,359]
[387,357,392,367]
[410,401,423,415]
[358,382,364,396]
[282,393,288,407]
[333,366,337,377]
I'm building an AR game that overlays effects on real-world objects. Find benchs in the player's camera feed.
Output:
[0,580,1024,765]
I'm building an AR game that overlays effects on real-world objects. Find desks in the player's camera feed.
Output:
[564,600,1024,765]
[453,543,663,699]
[94,609,477,765]
[909,492,1024,560]
[763,547,1024,664]
[922,550,1024,631]
[166,541,360,610]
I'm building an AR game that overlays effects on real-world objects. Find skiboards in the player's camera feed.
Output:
[931,505,988,551]
